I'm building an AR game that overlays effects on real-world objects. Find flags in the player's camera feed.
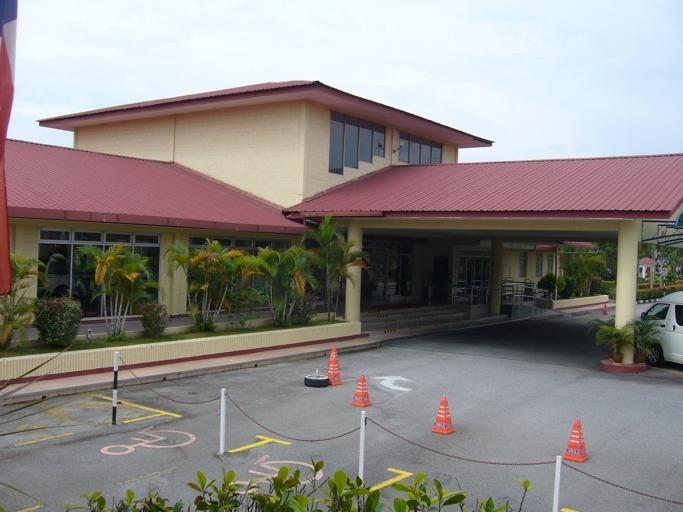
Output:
[0,0,19,296]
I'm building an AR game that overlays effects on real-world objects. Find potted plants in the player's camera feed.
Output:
[590,318,639,364]
[634,315,661,363]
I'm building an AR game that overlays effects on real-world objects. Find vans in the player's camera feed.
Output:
[45,252,151,298]
[637,290,683,366]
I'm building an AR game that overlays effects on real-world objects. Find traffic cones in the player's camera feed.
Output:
[562,418,590,463]
[350,374,372,408]
[328,345,343,387]
[431,396,456,435]
[601,303,609,315]
